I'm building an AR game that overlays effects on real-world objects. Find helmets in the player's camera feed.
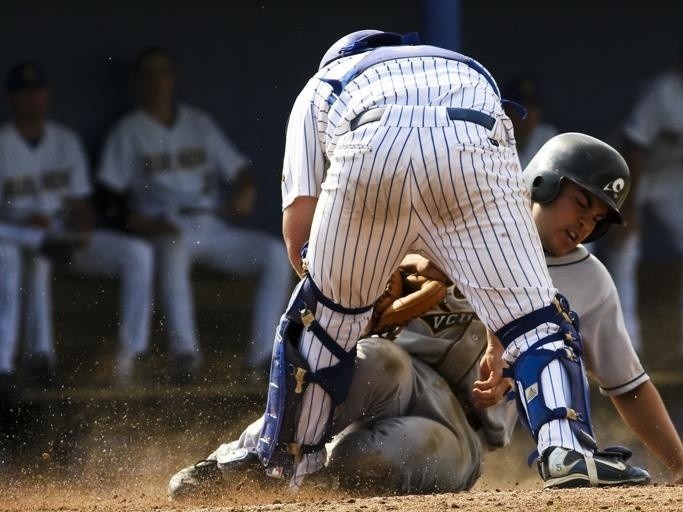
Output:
[317,29,401,68]
[521,132,632,243]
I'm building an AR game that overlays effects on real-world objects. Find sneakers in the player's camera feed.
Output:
[535,444,651,491]
[169,446,268,503]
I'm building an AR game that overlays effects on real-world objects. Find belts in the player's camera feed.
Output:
[351,106,494,129]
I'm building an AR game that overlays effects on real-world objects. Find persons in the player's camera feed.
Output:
[488,68,555,169]
[93,37,292,388]
[253,24,655,498]
[602,60,683,371]
[164,127,682,509]
[0,221,92,387]
[0,60,152,388]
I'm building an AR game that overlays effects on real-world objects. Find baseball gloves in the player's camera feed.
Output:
[370,254,453,330]
